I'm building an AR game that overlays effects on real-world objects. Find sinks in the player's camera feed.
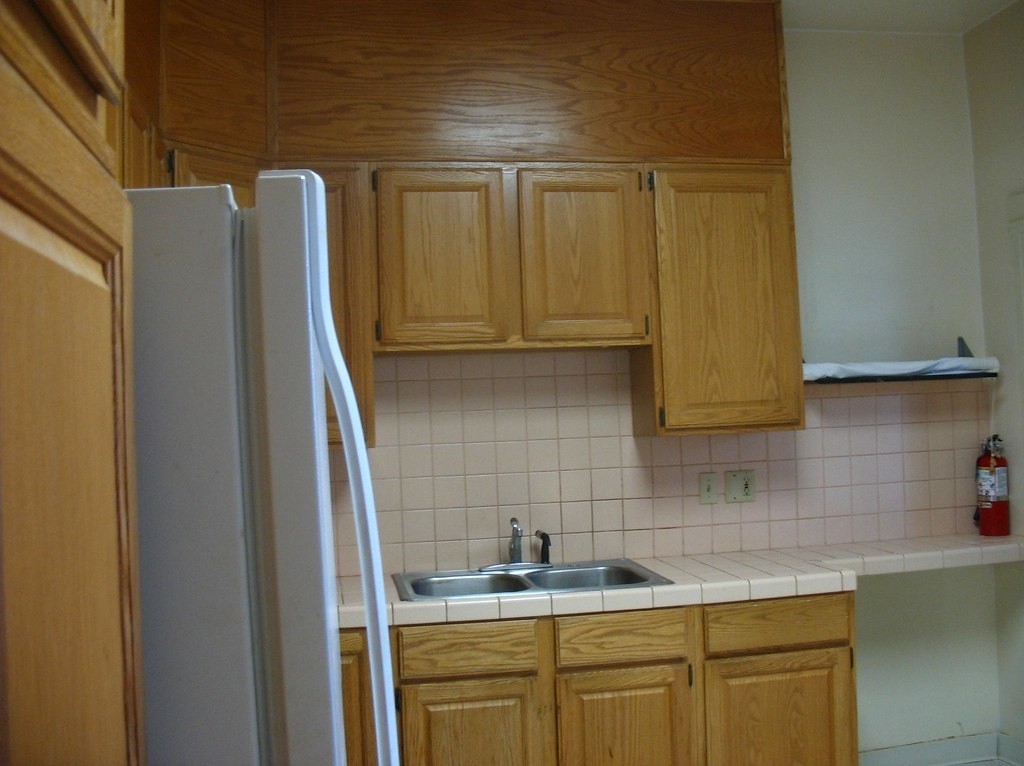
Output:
[528,564,646,589]
[411,572,532,595]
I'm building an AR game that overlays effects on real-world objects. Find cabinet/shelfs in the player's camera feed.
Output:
[0,48,146,766]
[163,142,804,441]
[337,591,858,766]
[30,0,126,105]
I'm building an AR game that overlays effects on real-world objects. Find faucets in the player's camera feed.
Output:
[509,518,523,561]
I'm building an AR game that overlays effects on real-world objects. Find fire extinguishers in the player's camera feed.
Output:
[972,434,1010,536]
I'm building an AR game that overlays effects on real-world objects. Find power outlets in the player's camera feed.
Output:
[725,470,755,503]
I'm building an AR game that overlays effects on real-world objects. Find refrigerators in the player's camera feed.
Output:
[122,169,400,766]
